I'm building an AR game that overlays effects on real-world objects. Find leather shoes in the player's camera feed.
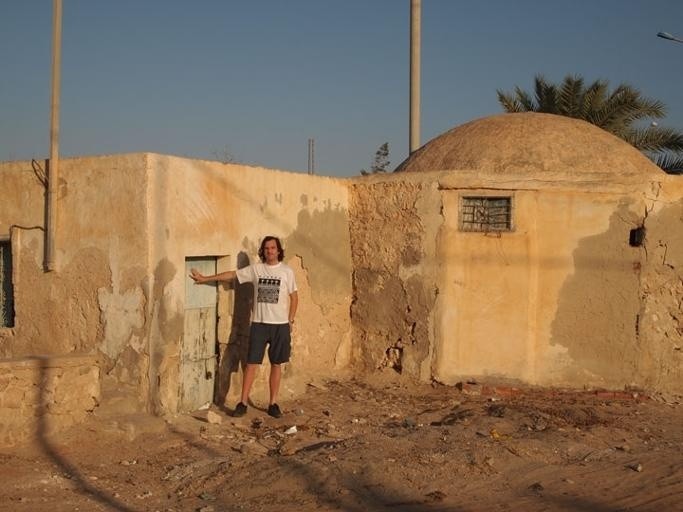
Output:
[231,402,247,417]
[268,403,282,418]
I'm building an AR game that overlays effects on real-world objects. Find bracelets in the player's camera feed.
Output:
[289,319,295,324]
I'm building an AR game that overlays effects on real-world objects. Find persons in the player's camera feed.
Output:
[189,236,299,418]
